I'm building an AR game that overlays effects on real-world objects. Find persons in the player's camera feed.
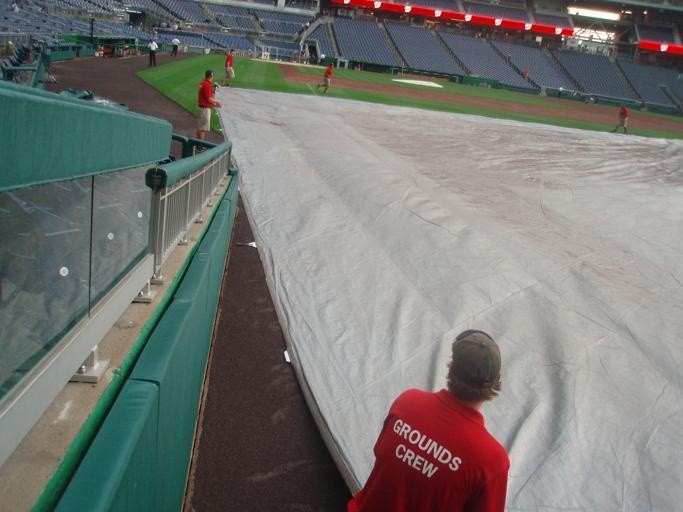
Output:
[153,27,158,41]
[170,37,180,57]
[147,38,159,67]
[222,48,236,86]
[523,66,529,79]
[317,63,334,93]
[346,329,511,512]
[195,70,221,152]
[590,95,599,104]
[611,102,631,134]
[559,84,563,96]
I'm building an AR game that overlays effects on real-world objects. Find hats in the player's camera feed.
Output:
[452,329,501,389]
[206,70,216,77]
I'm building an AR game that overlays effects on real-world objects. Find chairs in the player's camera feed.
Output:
[121,0,681,114]
[0,2,174,392]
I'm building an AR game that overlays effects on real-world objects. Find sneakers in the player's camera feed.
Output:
[195,146,207,153]
